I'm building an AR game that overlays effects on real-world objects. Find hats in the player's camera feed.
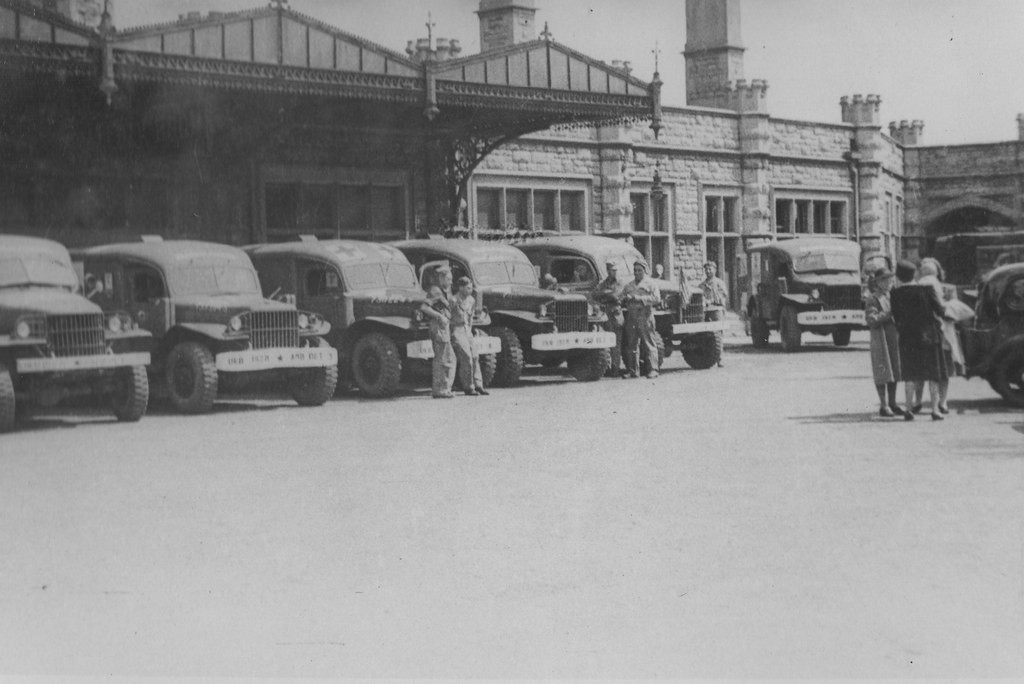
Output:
[457,276,471,286]
[633,258,648,275]
[895,260,917,273]
[703,261,717,267]
[871,268,894,282]
[436,267,451,274]
[606,261,618,270]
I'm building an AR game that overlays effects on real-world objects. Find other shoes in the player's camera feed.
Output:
[647,370,659,377]
[605,369,622,377]
[623,371,639,378]
[433,392,454,398]
[718,359,724,367]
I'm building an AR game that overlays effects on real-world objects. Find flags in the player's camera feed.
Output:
[680,268,691,310]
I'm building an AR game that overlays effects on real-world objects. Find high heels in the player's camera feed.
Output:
[912,403,923,414]
[932,413,945,420]
[465,387,490,396]
[938,402,948,414]
[905,411,914,420]
[880,405,905,417]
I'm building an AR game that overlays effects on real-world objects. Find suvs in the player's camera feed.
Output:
[512,227,728,373]
[71,232,342,414]
[3,230,152,436]
[397,235,619,382]
[743,222,873,348]
[969,262,1024,410]
[250,228,496,397]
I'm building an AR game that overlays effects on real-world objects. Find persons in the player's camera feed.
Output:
[591,261,627,376]
[866,256,975,421]
[448,276,490,395]
[618,260,662,378]
[699,262,727,366]
[421,267,457,396]
